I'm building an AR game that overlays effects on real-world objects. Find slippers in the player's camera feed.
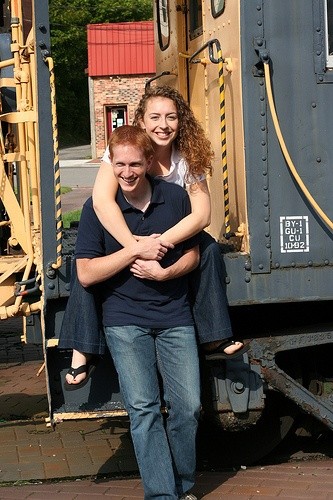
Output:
[201,339,252,361]
[64,359,94,391]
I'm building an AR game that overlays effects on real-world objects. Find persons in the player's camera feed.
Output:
[58,85,250,392]
[74,126,202,500]
